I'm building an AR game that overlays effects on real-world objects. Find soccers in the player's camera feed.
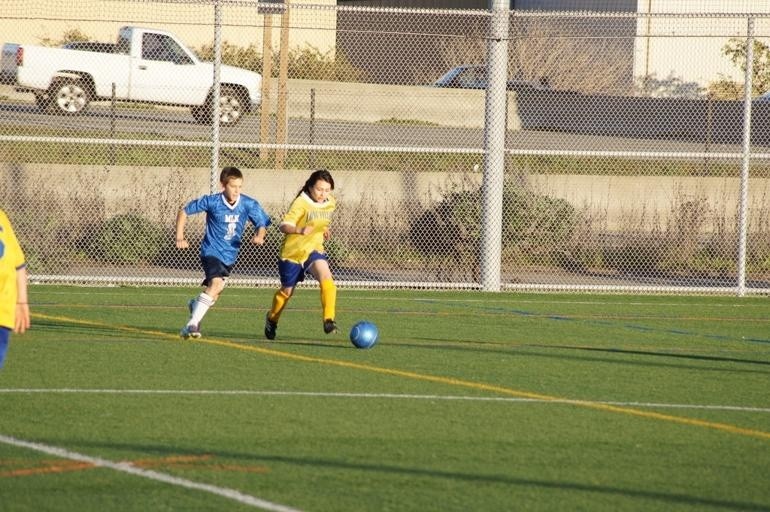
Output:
[350,322,379,348]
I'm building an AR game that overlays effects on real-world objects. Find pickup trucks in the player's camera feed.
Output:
[0,25,264,128]
[432,64,582,96]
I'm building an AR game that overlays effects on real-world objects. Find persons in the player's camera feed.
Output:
[265,169,339,341]
[176,166,272,340]
[0,205,33,371]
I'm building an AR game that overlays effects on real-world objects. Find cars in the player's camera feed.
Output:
[749,90,770,102]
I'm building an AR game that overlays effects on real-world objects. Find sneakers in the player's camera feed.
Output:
[180,300,202,339]
[265,310,277,339]
[324,318,338,335]
